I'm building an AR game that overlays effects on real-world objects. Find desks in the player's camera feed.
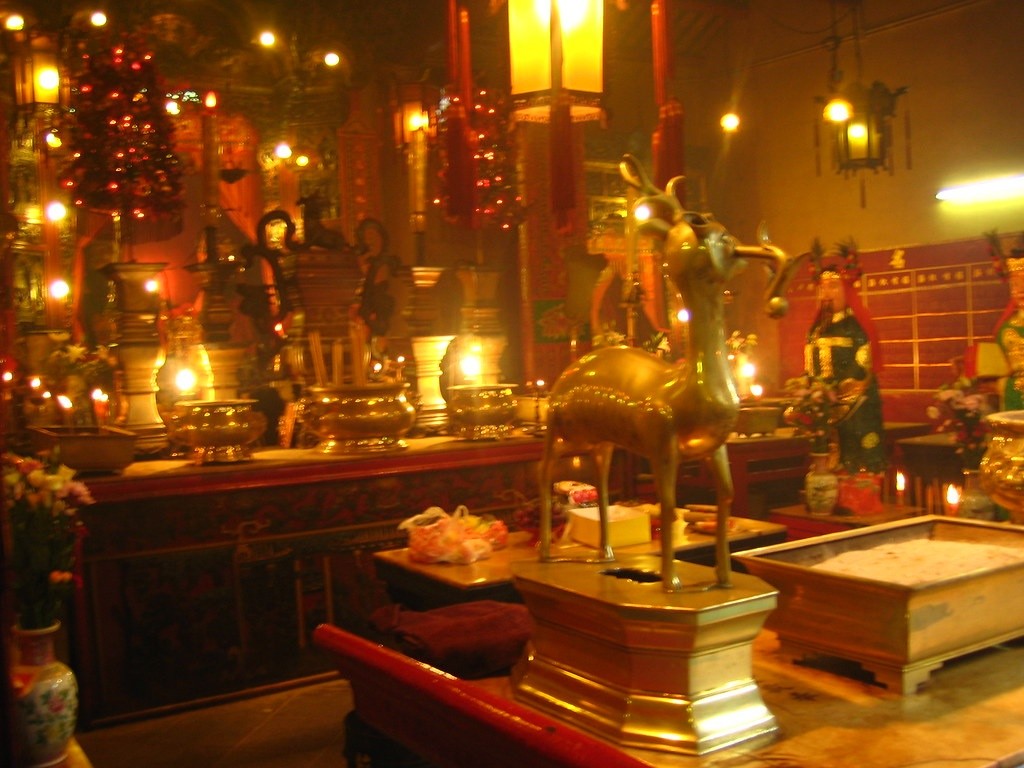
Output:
[626,422,933,520]
[0,424,546,732]
[894,432,962,486]
[372,505,790,610]
[313,621,1024,768]
[769,500,925,542]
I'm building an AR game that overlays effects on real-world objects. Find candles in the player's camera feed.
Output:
[947,483,959,517]
[895,470,906,510]
[57,389,112,433]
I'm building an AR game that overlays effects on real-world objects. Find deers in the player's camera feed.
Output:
[533,154,788,594]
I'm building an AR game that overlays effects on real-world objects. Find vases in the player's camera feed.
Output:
[803,452,840,517]
[0,618,81,768]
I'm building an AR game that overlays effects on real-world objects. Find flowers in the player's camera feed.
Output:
[785,371,853,452]
[0,452,97,631]
[926,388,989,449]
